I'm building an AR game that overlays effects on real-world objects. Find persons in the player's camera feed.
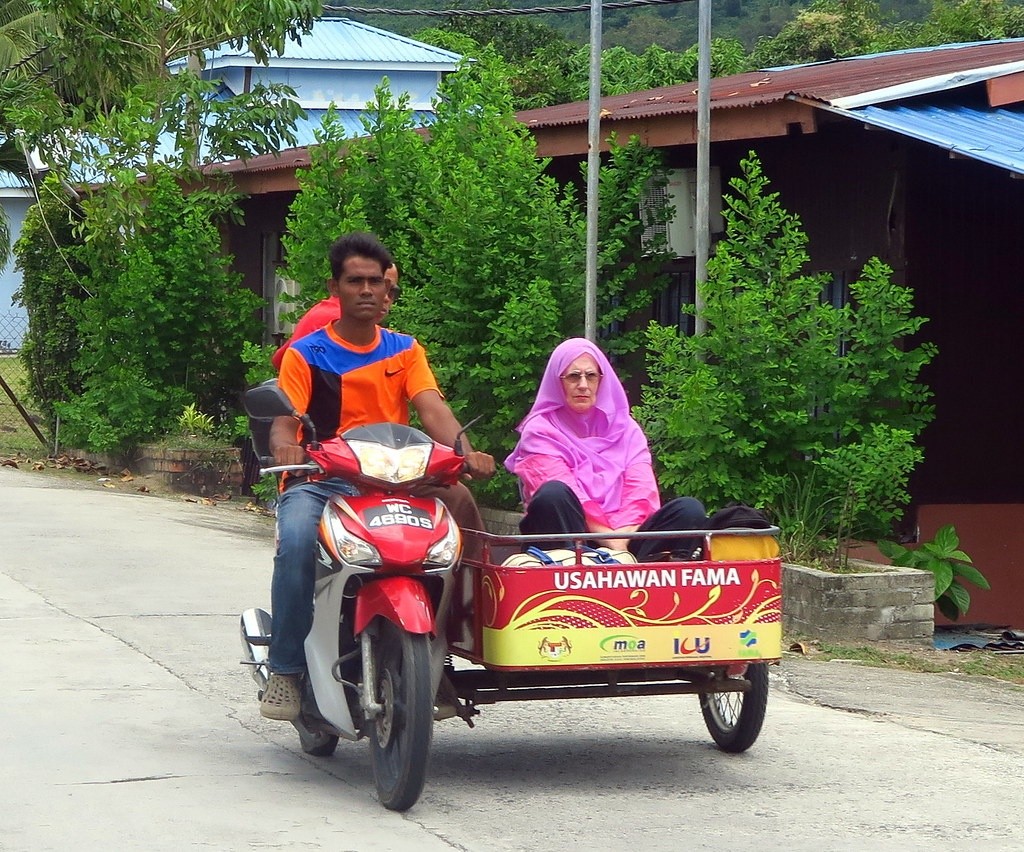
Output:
[271,258,489,563]
[258,233,497,723]
[503,337,708,557]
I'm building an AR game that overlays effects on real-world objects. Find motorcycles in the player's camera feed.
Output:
[238,377,789,813]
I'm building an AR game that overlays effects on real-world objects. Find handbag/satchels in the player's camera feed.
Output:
[501,542,640,571]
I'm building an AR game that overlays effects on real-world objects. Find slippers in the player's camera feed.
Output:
[259,670,305,723]
[431,677,456,722]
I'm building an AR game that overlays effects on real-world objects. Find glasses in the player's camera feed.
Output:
[381,276,403,301]
[559,371,604,384]
